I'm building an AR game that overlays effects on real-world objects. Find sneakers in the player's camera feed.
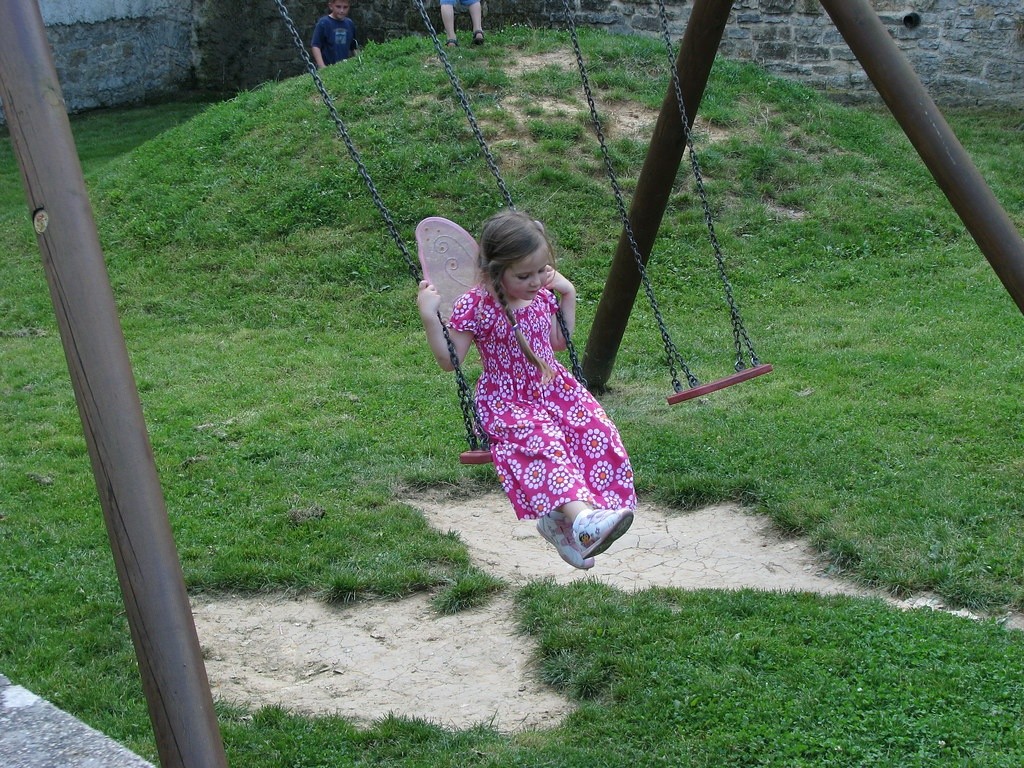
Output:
[571,508,634,560]
[536,511,595,569]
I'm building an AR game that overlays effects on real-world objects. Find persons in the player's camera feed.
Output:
[440,0,485,47]
[311,0,359,69]
[418,210,638,571]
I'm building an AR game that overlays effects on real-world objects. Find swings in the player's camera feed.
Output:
[558,0,773,406]
[276,0,587,464]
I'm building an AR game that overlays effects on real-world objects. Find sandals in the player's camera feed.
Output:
[446,38,459,48]
[473,29,484,43]
